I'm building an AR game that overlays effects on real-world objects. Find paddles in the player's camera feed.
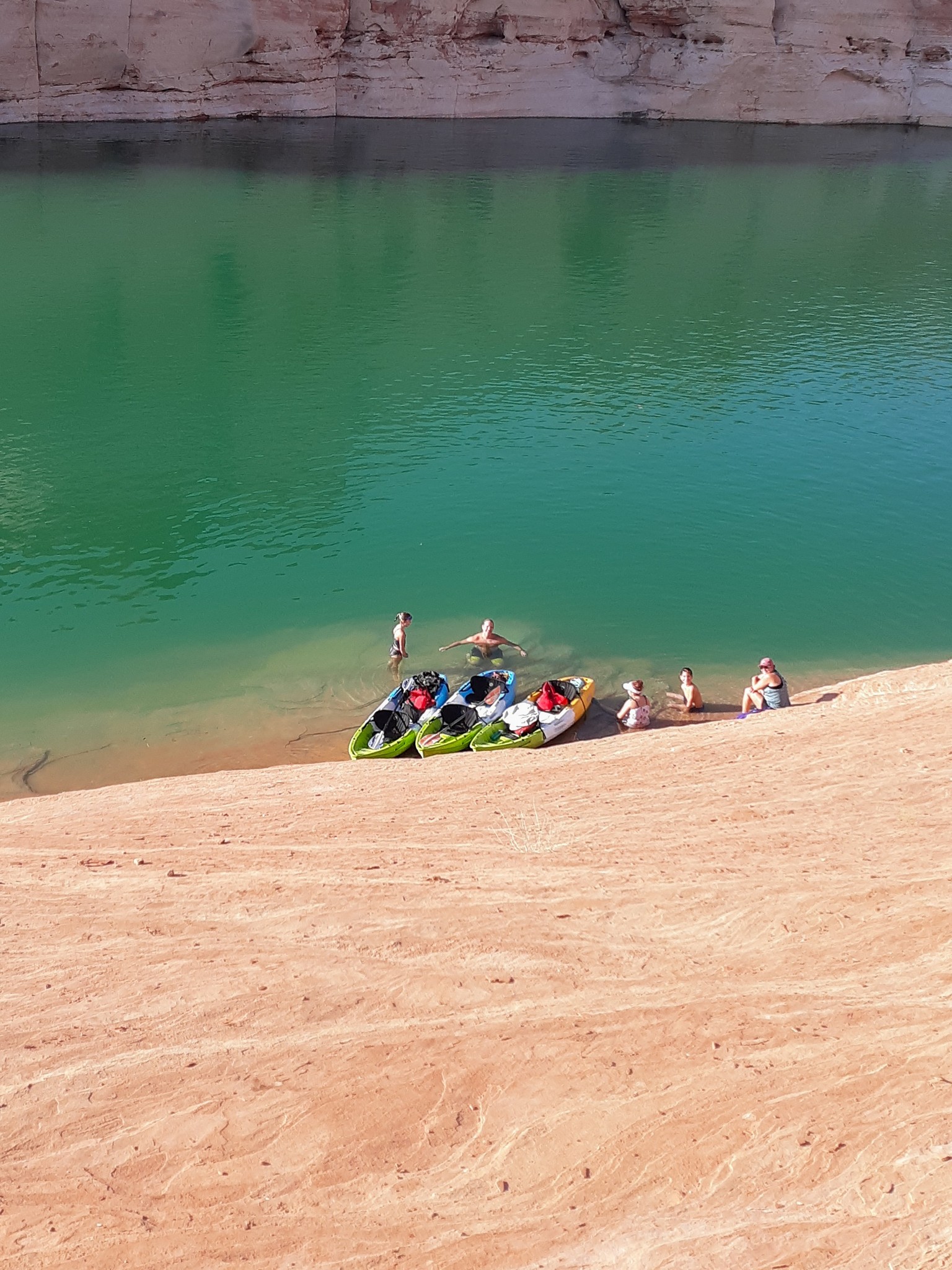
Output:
[367,680,413,750]
[418,686,501,747]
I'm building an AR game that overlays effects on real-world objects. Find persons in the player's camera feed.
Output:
[616,679,651,728]
[680,668,703,708]
[389,612,413,665]
[439,619,527,664]
[742,658,791,714]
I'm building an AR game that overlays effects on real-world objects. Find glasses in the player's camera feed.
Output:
[760,666,768,669]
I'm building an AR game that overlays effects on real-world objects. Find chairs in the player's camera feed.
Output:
[464,675,506,707]
[542,680,577,714]
[442,704,478,734]
[373,709,410,742]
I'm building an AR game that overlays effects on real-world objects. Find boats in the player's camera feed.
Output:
[416,669,516,760]
[468,675,596,752]
[348,671,449,760]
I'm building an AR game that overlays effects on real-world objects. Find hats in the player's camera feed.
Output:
[623,681,642,694]
[757,658,774,668]
